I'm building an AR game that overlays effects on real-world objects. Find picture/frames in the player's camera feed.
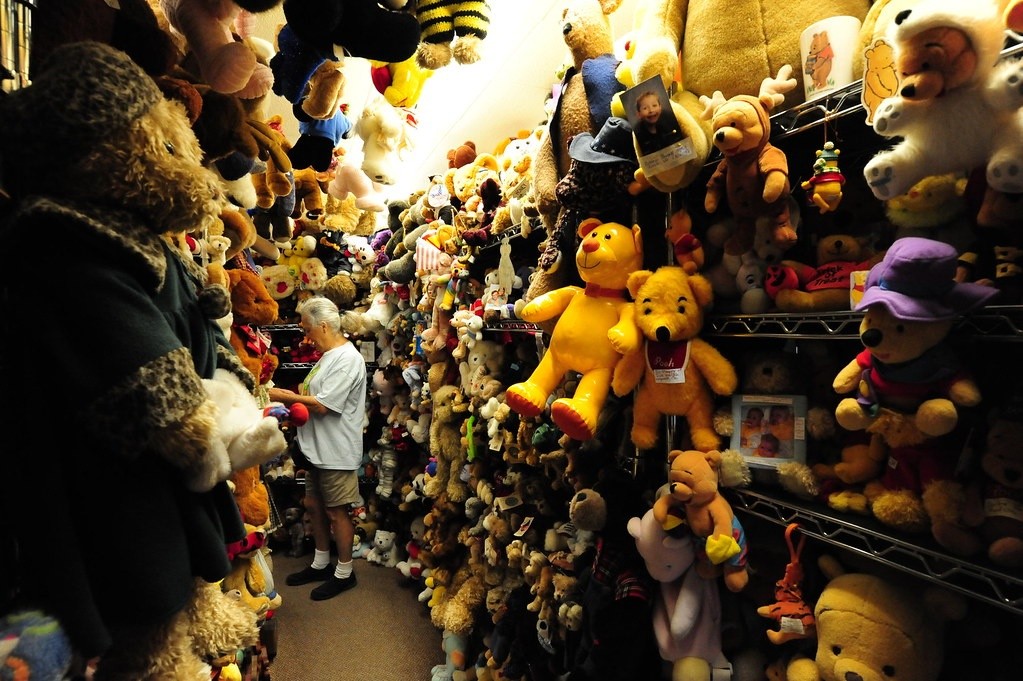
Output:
[729,393,808,472]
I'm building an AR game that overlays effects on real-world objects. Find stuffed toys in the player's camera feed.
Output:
[0,0,1022,681]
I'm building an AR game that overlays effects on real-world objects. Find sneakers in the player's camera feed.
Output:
[286,562,336,586]
[311,570,357,601]
